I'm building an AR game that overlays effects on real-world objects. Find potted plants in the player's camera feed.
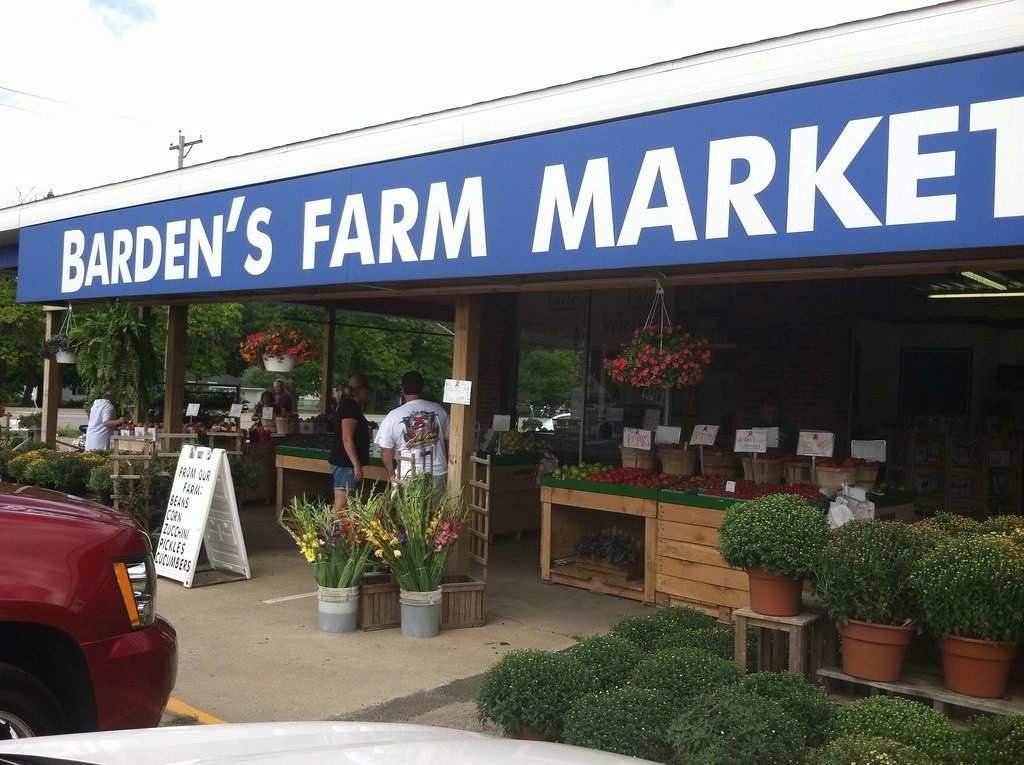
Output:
[0,413,264,560]
[473,493,1024,765]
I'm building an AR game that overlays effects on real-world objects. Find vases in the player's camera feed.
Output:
[399,585,443,639]
[316,584,360,633]
[262,352,295,372]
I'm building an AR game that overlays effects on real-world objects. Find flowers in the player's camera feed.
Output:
[239,326,320,369]
[273,469,410,589]
[602,323,712,388]
[330,468,473,591]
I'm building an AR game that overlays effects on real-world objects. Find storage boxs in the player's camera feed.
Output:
[121,430,135,437]
[134,427,163,442]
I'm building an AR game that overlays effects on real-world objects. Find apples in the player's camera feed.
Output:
[494,432,552,455]
[123,422,235,434]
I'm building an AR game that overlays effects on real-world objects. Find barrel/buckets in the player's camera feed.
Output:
[699,452,738,477]
[782,462,880,493]
[740,457,782,485]
[620,442,656,470]
[656,447,699,475]
[260,417,298,434]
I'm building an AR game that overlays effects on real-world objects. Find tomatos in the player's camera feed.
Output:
[662,443,854,468]
[552,462,680,489]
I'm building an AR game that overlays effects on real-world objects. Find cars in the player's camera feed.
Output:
[0,481,179,740]
[1,720,668,764]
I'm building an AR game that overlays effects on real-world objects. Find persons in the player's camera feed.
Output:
[379,370,450,511]
[251,389,282,425]
[329,373,377,513]
[273,378,294,413]
[83,382,126,452]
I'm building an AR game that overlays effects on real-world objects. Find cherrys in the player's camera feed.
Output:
[669,474,824,503]
